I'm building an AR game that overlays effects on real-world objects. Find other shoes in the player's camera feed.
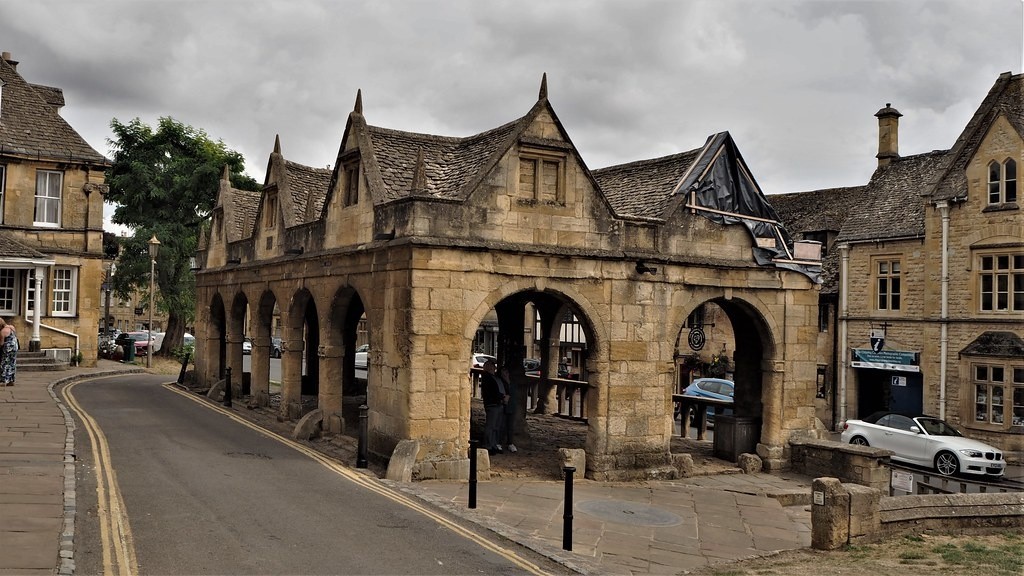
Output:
[495,448,503,454]
[6,381,15,386]
[488,449,497,455]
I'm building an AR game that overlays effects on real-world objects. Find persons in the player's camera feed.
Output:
[0,317,18,386]
[501,368,517,452]
[482,359,510,455]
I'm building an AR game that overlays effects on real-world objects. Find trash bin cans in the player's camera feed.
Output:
[121,338,137,362]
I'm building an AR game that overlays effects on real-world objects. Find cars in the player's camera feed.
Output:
[115,331,154,356]
[141,330,158,340]
[840,408,1007,478]
[243,337,252,354]
[525,361,573,400]
[270,336,281,358]
[354,343,369,369]
[114,328,122,339]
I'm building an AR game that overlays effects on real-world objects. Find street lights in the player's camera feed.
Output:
[147,233,161,367]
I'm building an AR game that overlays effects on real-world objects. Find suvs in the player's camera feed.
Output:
[679,377,734,426]
[473,353,497,384]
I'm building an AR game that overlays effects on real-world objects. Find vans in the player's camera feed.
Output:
[153,332,195,357]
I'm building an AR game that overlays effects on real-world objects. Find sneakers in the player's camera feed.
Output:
[508,443,518,453]
[496,444,503,449]
